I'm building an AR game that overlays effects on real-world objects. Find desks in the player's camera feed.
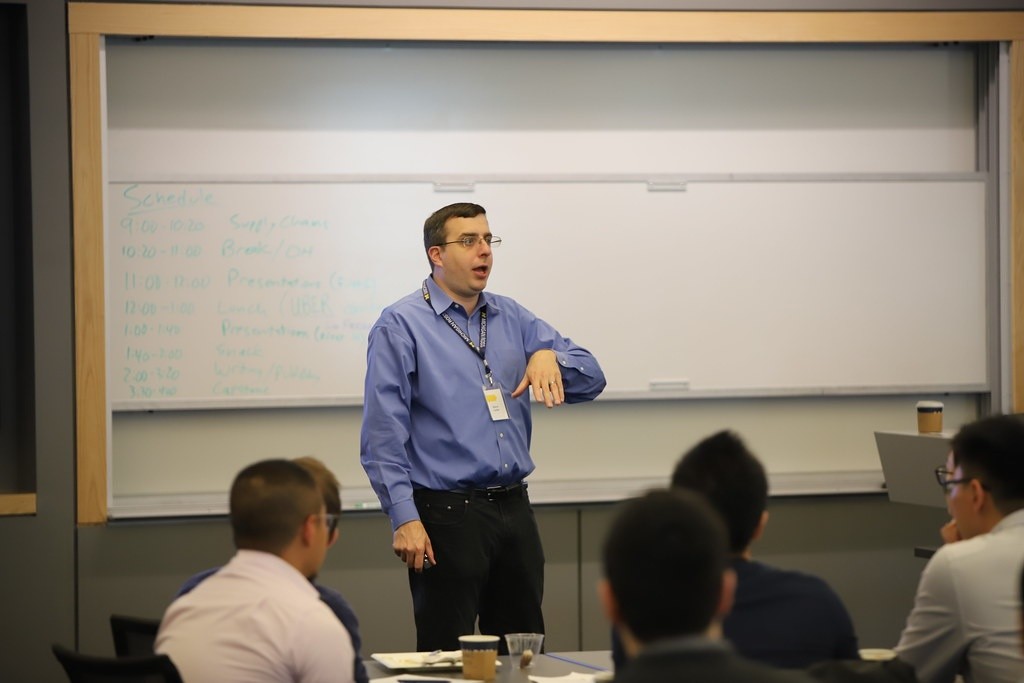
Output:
[361,651,615,683]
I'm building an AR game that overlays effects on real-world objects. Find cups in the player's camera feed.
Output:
[459,633,501,681]
[916,398,945,433]
[505,633,546,669]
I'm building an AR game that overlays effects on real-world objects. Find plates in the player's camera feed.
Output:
[371,649,463,672]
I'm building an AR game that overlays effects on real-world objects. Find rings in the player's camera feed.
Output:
[550,381,555,385]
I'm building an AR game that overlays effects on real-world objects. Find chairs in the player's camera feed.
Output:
[110,612,163,658]
[49,641,185,683]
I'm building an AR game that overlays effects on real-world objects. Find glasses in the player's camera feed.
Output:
[435,235,502,248]
[936,464,954,484]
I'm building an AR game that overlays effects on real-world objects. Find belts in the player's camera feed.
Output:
[449,481,529,499]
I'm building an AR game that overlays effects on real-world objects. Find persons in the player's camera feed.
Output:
[614,429,861,668]
[592,488,808,680]
[175,455,369,682]
[151,459,354,683]
[895,414,1023,683]
[360,202,607,657]
